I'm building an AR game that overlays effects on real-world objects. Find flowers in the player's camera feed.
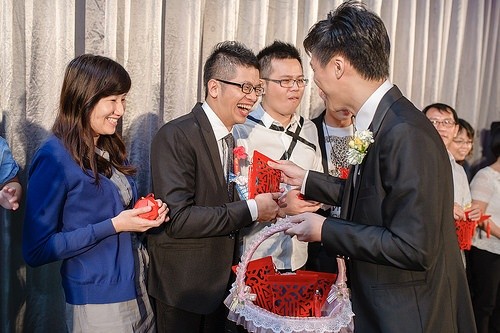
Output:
[233,146,248,161]
[346,129,374,166]
[339,167,350,180]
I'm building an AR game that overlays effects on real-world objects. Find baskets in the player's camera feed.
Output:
[223,219,355,333]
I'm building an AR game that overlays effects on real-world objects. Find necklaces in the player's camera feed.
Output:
[324,120,355,167]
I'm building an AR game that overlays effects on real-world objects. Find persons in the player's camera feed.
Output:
[147,42,287,333]
[230,40,500,333]
[0,135,21,211]
[22,54,170,333]
[269,1,479,333]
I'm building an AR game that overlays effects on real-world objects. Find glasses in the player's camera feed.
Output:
[214,78,264,96]
[453,139,474,145]
[261,78,308,88]
[430,119,456,128]
